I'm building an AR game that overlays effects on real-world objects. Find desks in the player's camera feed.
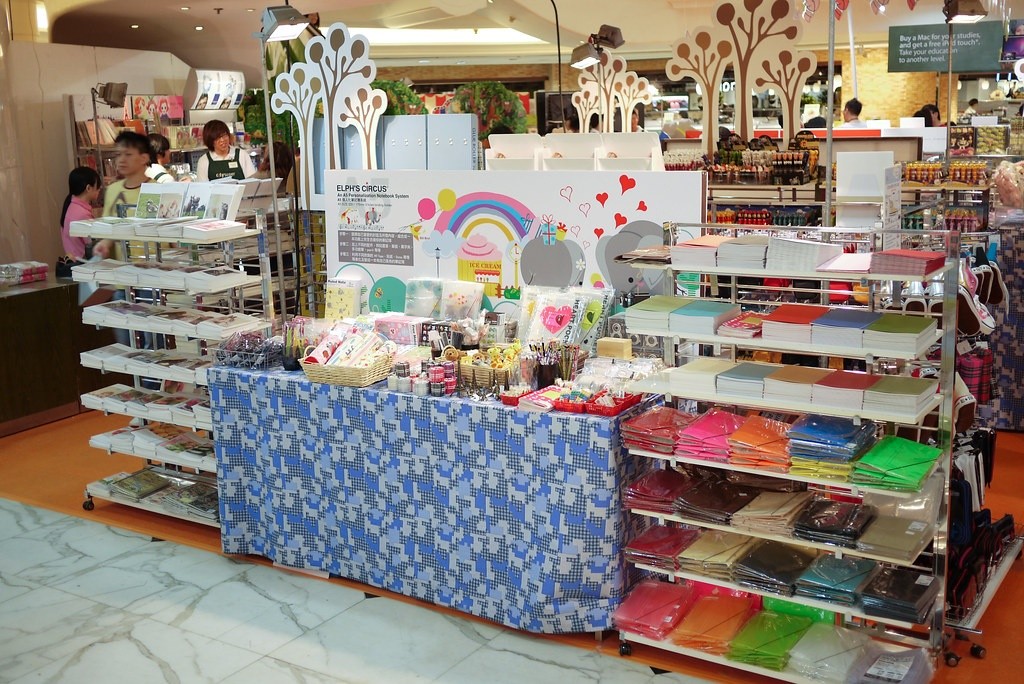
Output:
[0,272,134,438]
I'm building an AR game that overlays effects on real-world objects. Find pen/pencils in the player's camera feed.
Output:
[529,337,581,383]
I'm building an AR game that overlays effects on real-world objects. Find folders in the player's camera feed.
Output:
[613,407,945,684]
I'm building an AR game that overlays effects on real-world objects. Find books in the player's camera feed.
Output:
[65,93,309,528]
[607,230,973,684]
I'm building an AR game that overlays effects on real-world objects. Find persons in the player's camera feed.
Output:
[960,99,980,123]
[779,97,868,130]
[60,167,112,316]
[144,133,194,183]
[196,119,256,182]
[912,103,956,127]
[487,104,690,135]
[92,130,178,427]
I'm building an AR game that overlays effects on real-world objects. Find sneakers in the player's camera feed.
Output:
[920,365,976,433]
[881,242,1008,337]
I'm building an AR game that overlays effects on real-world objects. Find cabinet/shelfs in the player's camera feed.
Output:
[69,146,1024,684]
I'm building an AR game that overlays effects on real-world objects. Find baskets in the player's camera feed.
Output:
[435,345,518,389]
[500,390,535,406]
[210,342,284,370]
[298,345,396,387]
[553,389,644,417]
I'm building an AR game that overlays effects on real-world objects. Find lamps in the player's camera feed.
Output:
[99,82,128,108]
[595,24,625,48]
[261,5,310,43]
[945,0,988,24]
[570,43,600,70]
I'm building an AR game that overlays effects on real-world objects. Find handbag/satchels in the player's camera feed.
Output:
[946,508,1016,619]
